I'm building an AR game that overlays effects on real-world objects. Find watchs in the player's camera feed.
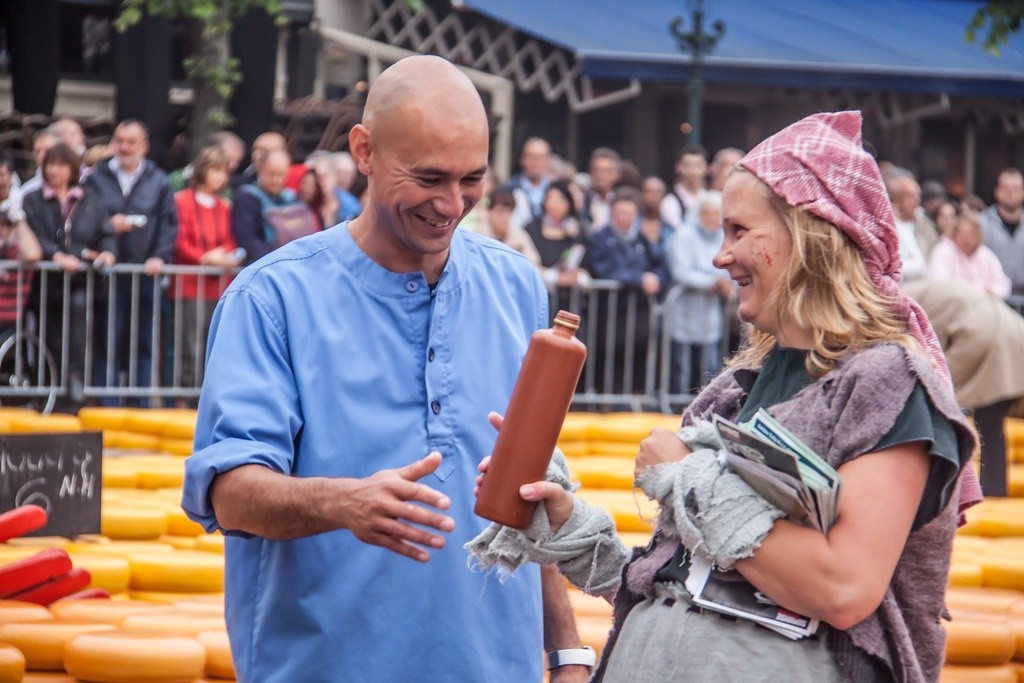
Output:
[546,646,596,671]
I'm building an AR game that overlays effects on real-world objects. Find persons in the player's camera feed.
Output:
[874,154,1023,497]
[459,137,759,416]
[0,114,369,410]
[477,107,984,683]
[181,55,595,683]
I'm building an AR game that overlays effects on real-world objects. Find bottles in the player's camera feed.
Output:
[474,310,587,529]
